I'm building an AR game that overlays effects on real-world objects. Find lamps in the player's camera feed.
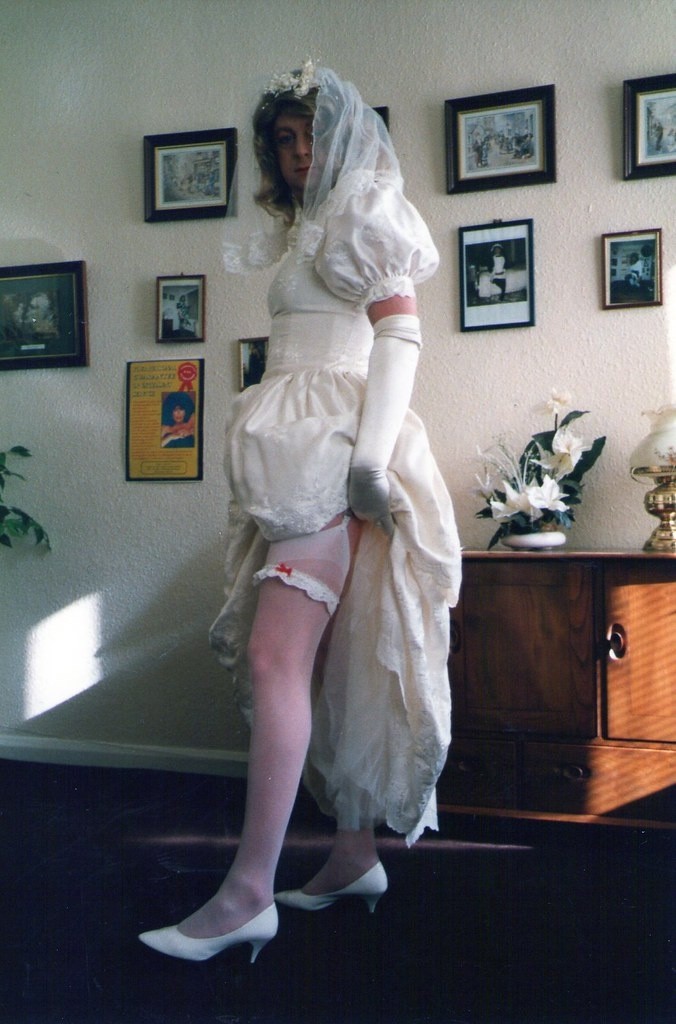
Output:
[628,403,676,552]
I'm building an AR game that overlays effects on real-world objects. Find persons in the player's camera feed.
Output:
[489,244,506,300]
[625,251,642,290]
[160,399,195,448]
[176,295,186,333]
[655,121,663,149]
[135,60,444,964]
[475,122,535,168]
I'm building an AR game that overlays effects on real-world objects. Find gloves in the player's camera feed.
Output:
[348,316,424,537]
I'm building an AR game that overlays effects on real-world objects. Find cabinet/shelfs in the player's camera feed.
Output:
[422,551,676,858]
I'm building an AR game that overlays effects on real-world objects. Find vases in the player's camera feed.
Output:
[500,532,566,551]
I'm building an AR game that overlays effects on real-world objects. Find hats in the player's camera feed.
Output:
[492,244,503,249]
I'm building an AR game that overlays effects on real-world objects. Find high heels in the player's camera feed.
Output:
[274,861,388,913]
[138,902,278,963]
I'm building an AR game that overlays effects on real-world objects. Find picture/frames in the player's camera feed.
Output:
[143,127,237,224]
[238,336,270,392]
[458,218,536,333]
[444,84,557,195]
[622,74,676,180]
[601,228,663,310]
[155,274,206,343]
[0,260,91,372]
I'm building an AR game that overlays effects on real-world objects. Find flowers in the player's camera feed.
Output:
[258,45,322,110]
[471,386,607,551]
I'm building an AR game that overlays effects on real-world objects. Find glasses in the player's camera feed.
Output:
[30,304,37,308]
